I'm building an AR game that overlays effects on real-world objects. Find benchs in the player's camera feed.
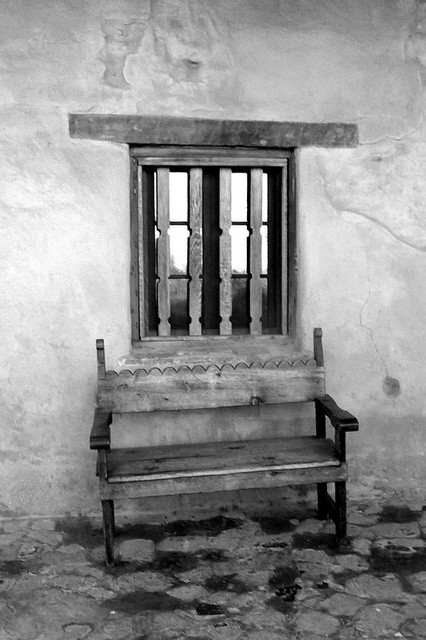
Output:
[90,323,360,565]
